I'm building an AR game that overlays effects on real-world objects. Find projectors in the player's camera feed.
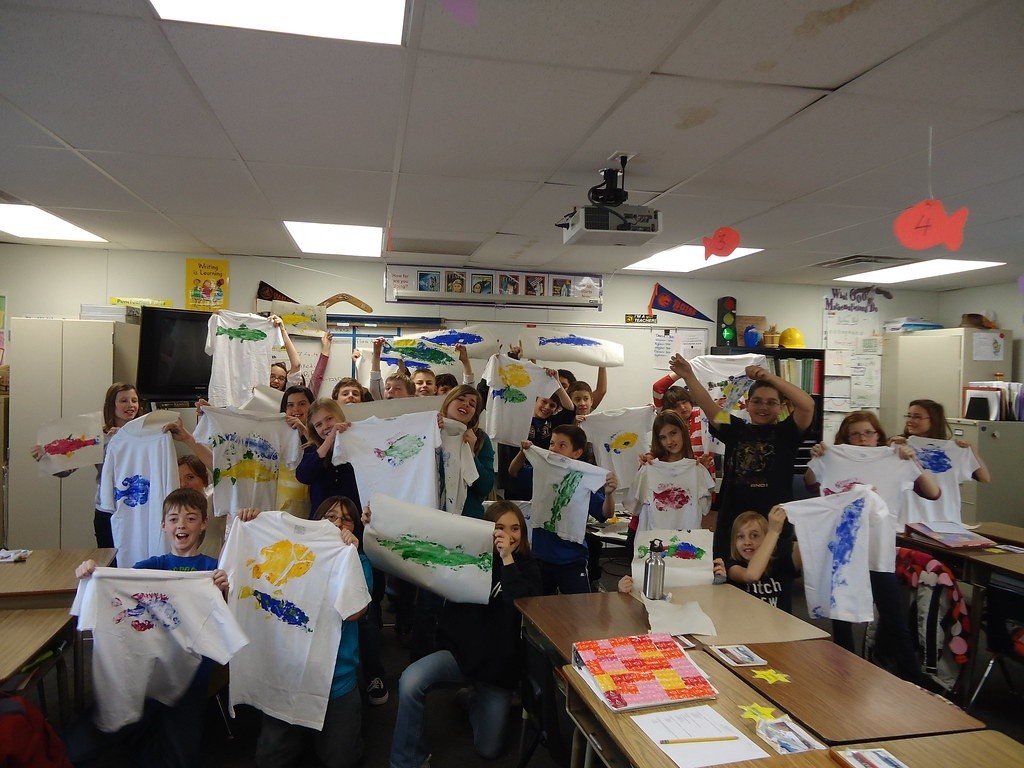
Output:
[563,205,663,246]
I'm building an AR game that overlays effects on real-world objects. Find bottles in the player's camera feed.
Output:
[643,538,666,600]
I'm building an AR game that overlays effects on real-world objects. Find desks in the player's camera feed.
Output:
[511,580,1024,768]
[0,542,118,739]
[896,521,1024,714]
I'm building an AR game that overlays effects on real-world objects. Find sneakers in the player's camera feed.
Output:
[365,668,389,705]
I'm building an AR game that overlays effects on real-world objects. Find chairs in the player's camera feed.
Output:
[863,544,970,705]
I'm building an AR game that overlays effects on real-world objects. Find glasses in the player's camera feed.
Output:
[904,414,930,422]
[750,399,779,407]
[270,375,285,384]
[850,430,878,439]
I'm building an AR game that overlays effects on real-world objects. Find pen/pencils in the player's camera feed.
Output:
[660,736,740,744]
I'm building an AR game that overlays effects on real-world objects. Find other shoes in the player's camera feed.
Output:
[394,621,414,647]
[451,687,478,711]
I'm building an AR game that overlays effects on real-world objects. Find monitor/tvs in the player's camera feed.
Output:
[136,306,217,402]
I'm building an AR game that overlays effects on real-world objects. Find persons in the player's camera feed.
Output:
[29,309,992,768]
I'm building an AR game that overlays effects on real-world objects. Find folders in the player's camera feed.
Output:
[903,521,998,552]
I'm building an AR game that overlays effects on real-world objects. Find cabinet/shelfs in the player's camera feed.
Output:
[8,316,115,550]
[879,329,1013,437]
[711,347,825,473]
